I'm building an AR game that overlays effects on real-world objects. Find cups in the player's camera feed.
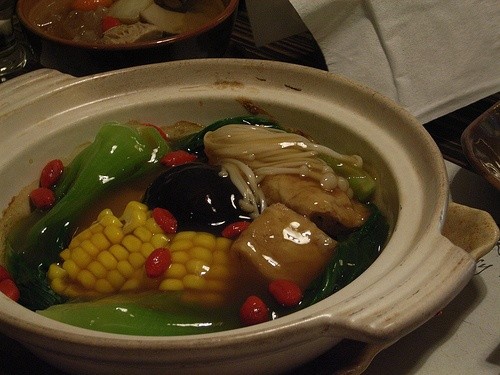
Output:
[1,0,30,84]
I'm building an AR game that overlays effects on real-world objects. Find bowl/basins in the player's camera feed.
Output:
[461,96,499,189]
[15,1,239,80]
[0,57,478,375]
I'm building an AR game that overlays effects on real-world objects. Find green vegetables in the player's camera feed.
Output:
[8,121,169,314]
[34,200,390,336]
[170,115,375,200]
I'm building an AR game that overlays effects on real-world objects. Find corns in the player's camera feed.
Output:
[155,231,233,306]
[47,200,172,297]
